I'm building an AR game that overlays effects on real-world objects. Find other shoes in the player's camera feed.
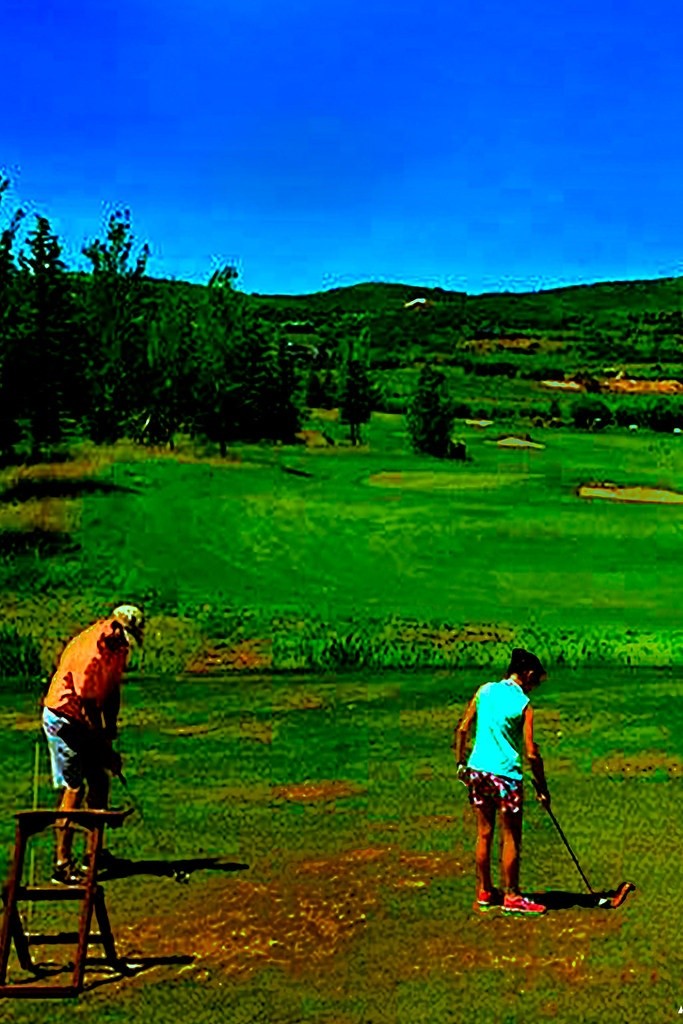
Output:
[81,848,133,871]
[49,861,89,887]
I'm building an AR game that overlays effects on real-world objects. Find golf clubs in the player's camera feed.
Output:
[118,774,191,883]
[533,780,611,908]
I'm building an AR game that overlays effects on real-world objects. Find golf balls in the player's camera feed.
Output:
[556,730,563,738]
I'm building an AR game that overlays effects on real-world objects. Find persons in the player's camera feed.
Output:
[40,603,144,888]
[455,647,549,913]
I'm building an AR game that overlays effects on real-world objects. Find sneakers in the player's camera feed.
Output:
[477,887,505,906]
[502,892,547,914]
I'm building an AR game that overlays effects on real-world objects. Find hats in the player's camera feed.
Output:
[114,605,144,647]
